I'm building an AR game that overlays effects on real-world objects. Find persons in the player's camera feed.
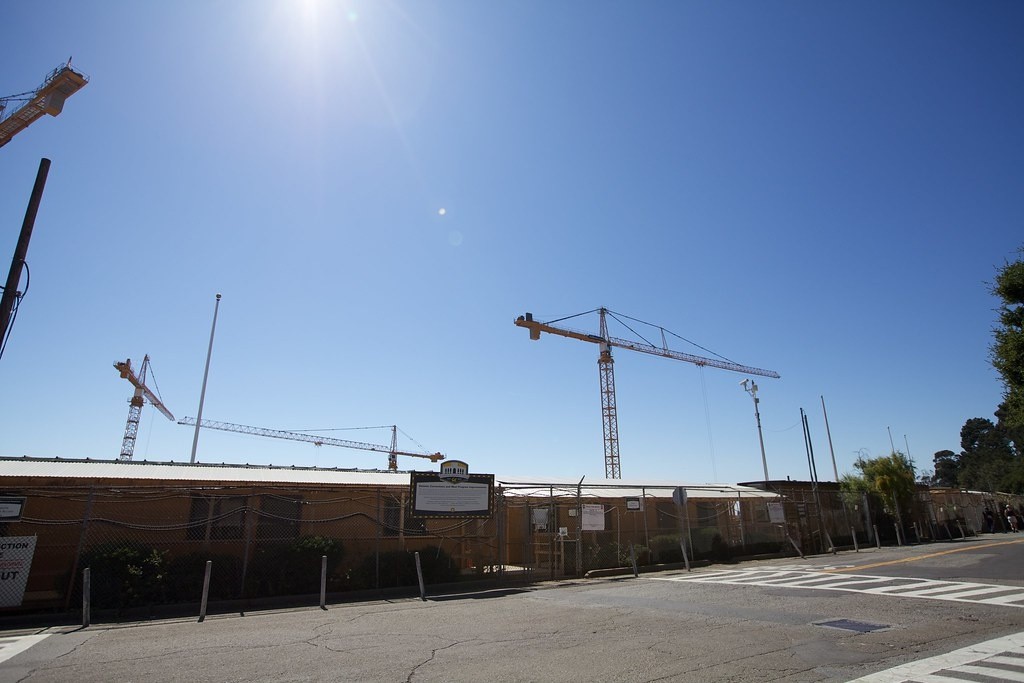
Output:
[982,508,996,535]
[1005,505,1020,532]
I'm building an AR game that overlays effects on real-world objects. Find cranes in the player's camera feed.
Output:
[0,56,91,146]
[114,353,175,461]
[177,416,445,470]
[513,306,781,478]
